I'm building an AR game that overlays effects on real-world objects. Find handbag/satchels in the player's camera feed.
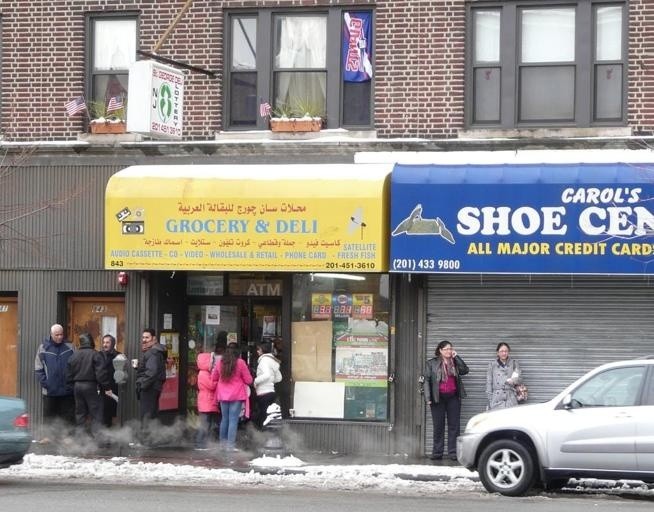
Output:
[512,360,528,402]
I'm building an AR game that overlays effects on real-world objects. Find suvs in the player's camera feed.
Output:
[455,356,654,502]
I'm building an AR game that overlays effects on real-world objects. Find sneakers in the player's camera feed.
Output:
[195,446,244,454]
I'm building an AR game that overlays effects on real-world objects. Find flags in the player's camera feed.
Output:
[341,12,373,84]
[64,95,88,116]
[260,97,272,117]
[106,93,125,112]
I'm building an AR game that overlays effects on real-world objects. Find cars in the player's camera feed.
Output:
[0,395,29,471]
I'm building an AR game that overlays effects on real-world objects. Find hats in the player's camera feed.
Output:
[78,333,95,348]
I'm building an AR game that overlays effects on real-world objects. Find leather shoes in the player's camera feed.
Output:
[430,455,442,460]
[450,455,456,460]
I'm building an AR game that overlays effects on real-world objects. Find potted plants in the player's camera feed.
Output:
[269,99,323,132]
[86,97,127,134]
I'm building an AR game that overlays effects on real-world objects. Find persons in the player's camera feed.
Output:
[251,343,284,428]
[485,341,524,411]
[210,341,254,450]
[194,350,220,450]
[64,332,113,441]
[99,334,124,429]
[33,324,77,445]
[126,326,170,450]
[424,340,471,463]
